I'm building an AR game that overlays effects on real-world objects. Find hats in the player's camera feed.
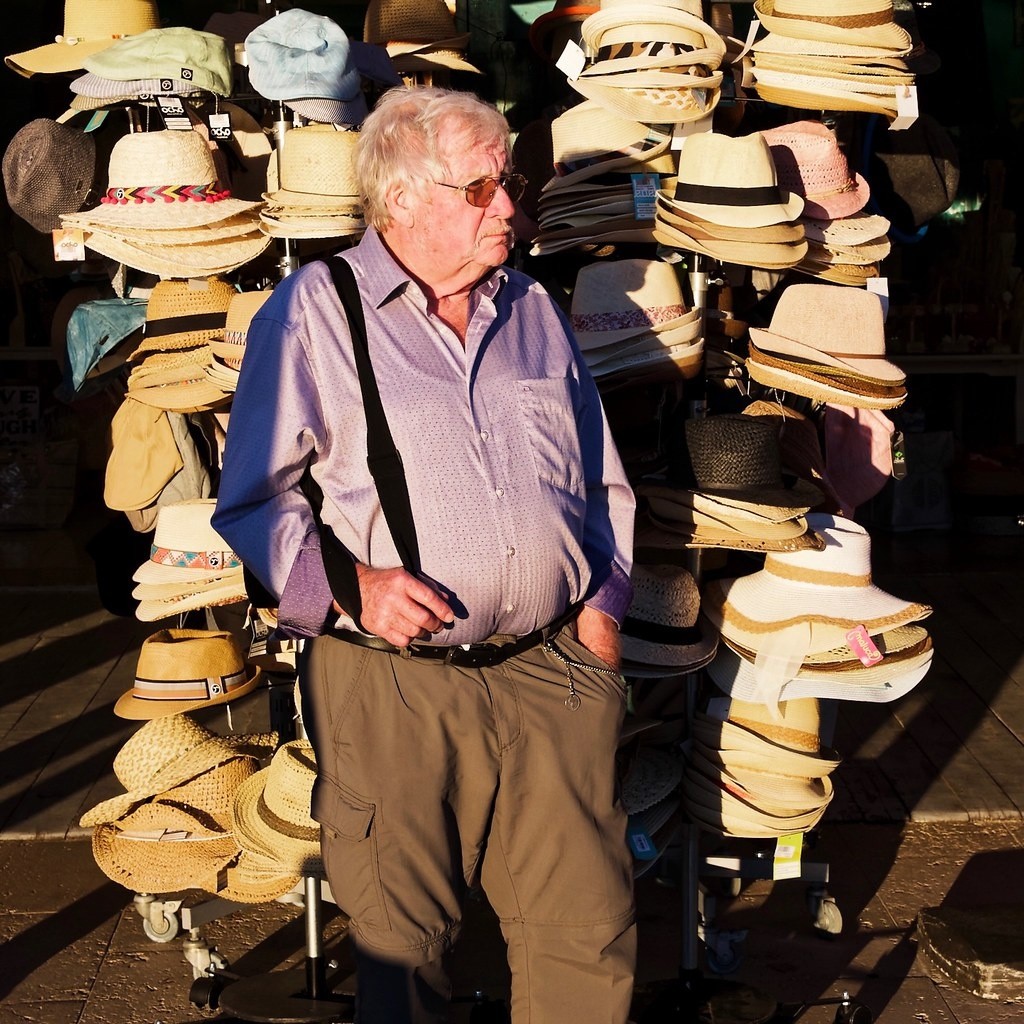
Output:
[3,0,923,900]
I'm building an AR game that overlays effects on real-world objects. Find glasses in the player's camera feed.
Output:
[429,174,529,208]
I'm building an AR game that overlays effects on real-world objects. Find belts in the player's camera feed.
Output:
[318,600,583,669]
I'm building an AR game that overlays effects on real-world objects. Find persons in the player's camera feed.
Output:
[210,78,651,1024]
